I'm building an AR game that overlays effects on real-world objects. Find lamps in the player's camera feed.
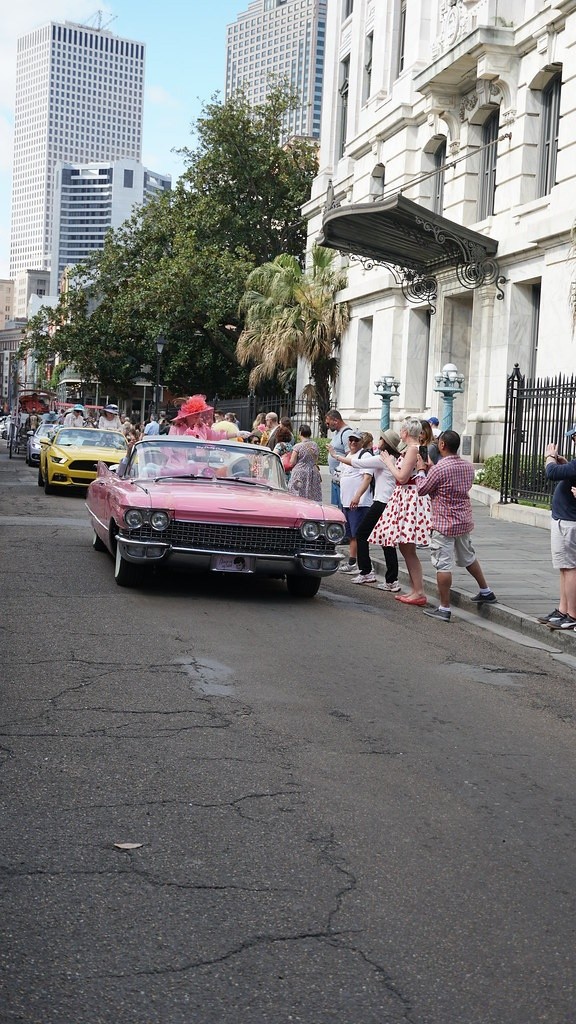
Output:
[432,363,465,435]
[373,374,400,434]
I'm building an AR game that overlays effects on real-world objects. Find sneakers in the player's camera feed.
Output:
[537,610,566,624]
[351,571,377,584]
[469,590,497,603]
[337,564,360,575]
[377,581,402,591]
[546,613,576,630]
[422,607,451,622]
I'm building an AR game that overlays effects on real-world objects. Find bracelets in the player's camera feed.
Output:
[417,468,427,474]
[546,454,557,461]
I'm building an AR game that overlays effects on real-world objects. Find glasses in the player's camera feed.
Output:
[572,436,576,442]
[249,440,260,444]
[348,437,360,442]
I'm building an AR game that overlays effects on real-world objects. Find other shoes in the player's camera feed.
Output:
[395,592,427,606]
[417,542,432,549]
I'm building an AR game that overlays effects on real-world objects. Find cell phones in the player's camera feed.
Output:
[419,446,428,466]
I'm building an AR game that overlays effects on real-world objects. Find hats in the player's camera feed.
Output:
[169,396,215,422]
[347,430,364,441]
[429,417,439,427]
[43,411,60,422]
[566,425,576,436]
[74,404,84,411]
[102,403,119,415]
[380,427,402,454]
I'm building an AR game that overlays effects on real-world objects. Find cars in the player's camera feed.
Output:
[84,435,347,598]
[26,423,132,495]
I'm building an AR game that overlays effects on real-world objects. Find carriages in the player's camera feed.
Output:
[0,389,64,458]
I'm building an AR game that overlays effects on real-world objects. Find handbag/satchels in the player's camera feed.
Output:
[278,442,295,472]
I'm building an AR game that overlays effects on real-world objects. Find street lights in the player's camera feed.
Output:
[433,363,465,433]
[153,331,166,414]
[373,375,401,432]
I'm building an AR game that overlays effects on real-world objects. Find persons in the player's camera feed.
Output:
[98,404,124,447]
[157,411,172,435]
[537,424,576,632]
[326,410,444,604]
[26,393,42,414]
[289,424,322,505]
[120,412,146,444]
[144,413,159,436]
[253,412,294,487]
[214,411,260,446]
[42,403,98,428]
[0,406,11,422]
[168,393,252,457]
[227,453,252,478]
[411,430,498,622]
